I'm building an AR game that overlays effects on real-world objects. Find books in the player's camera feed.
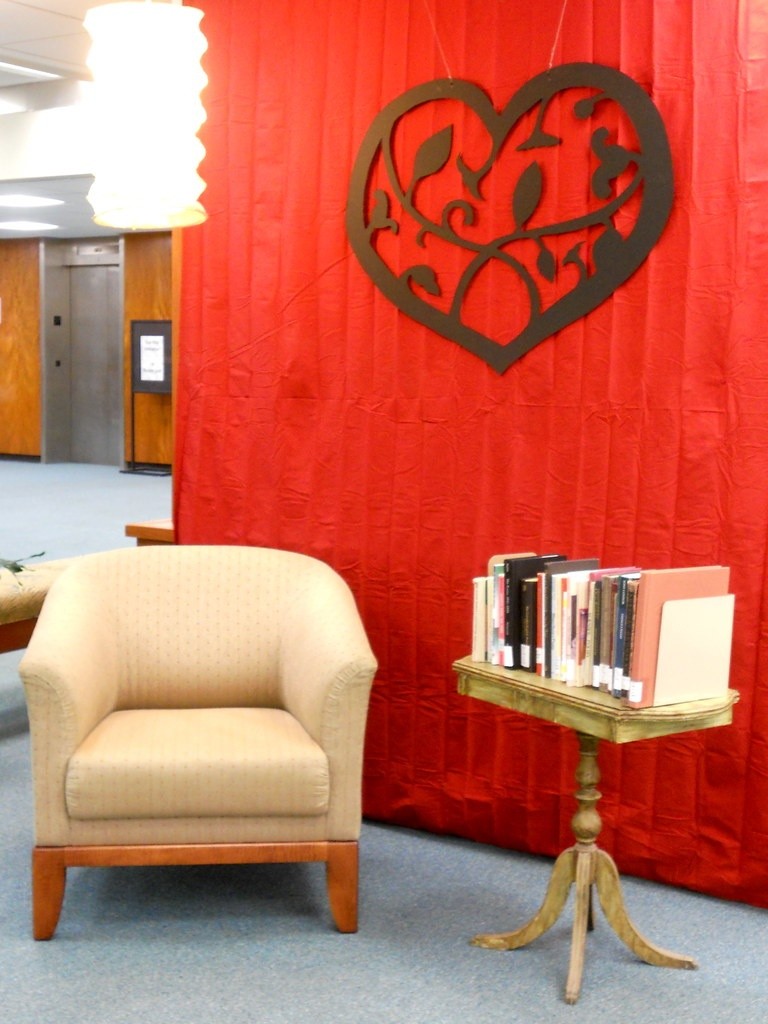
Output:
[472,551,735,709]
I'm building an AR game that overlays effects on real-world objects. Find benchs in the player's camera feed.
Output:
[0,552,94,654]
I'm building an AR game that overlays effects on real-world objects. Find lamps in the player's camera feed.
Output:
[84,0,210,231]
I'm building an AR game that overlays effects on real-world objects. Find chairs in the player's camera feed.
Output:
[17,546,377,939]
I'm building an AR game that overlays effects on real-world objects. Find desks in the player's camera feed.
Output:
[452,654,740,1005]
